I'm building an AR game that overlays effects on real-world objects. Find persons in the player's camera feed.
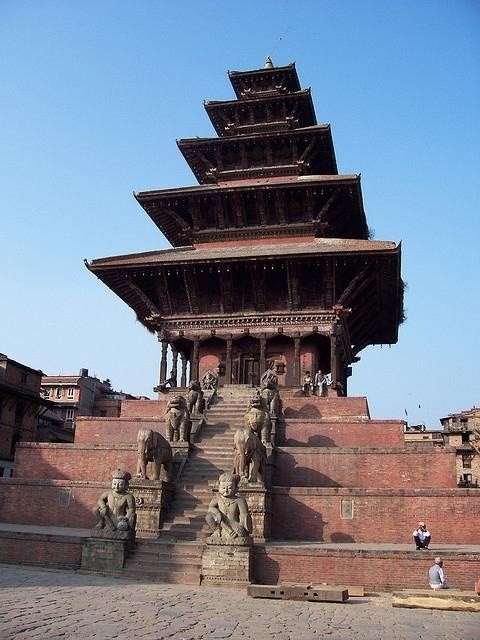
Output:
[94,471,136,531]
[205,471,253,538]
[428,556,450,589]
[161,370,177,388]
[314,369,330,398]
[302,370,314,394]
[413,522,431,549]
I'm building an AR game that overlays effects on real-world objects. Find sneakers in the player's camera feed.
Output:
[416,546,428,550]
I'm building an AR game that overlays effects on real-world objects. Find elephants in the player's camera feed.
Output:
[136,426,173,481]
[232,427,267,483]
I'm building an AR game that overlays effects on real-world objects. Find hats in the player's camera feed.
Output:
[419,521,425,526]
